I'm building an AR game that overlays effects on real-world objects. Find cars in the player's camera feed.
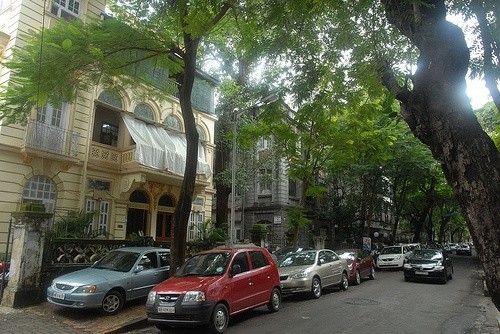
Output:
[277,249,350,298]
[47,247,170,316]
[335,248,376,285]
[145,246,281,334]
[403,248,453,284]
[402,242,473,255]
[274,246,314,261]
[377,246,412,271]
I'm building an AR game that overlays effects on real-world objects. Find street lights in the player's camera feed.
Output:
[230,94,280,242]
